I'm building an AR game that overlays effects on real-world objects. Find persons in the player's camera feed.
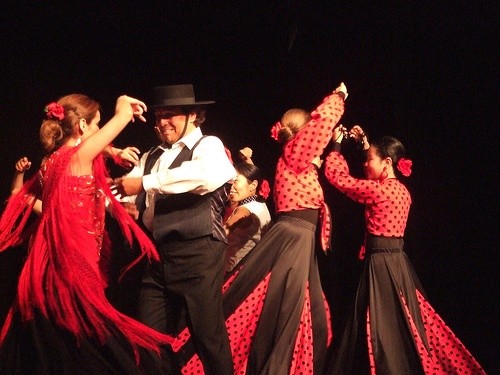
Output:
[324,117,484,375]
[0,81,349,374]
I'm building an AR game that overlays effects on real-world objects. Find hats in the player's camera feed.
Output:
[145,83,215,107]
